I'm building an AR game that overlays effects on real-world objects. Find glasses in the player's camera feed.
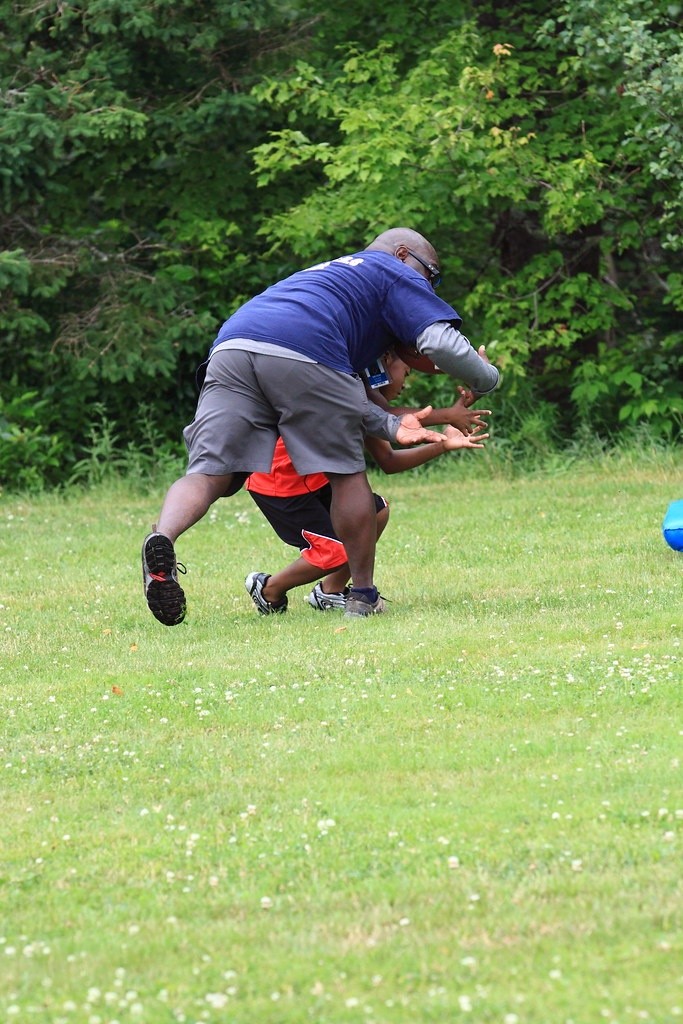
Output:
[408,249,441,290]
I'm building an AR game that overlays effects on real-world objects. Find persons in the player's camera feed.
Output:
[142,228,498,625]
[244,340,492,615]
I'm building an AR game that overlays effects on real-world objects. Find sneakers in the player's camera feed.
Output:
[143,532,187,626]
[307,583,349,611]
[345,585,387,617]
[245,572,288,614]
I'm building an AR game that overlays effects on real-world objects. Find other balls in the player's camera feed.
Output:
[662,498,682,552]
